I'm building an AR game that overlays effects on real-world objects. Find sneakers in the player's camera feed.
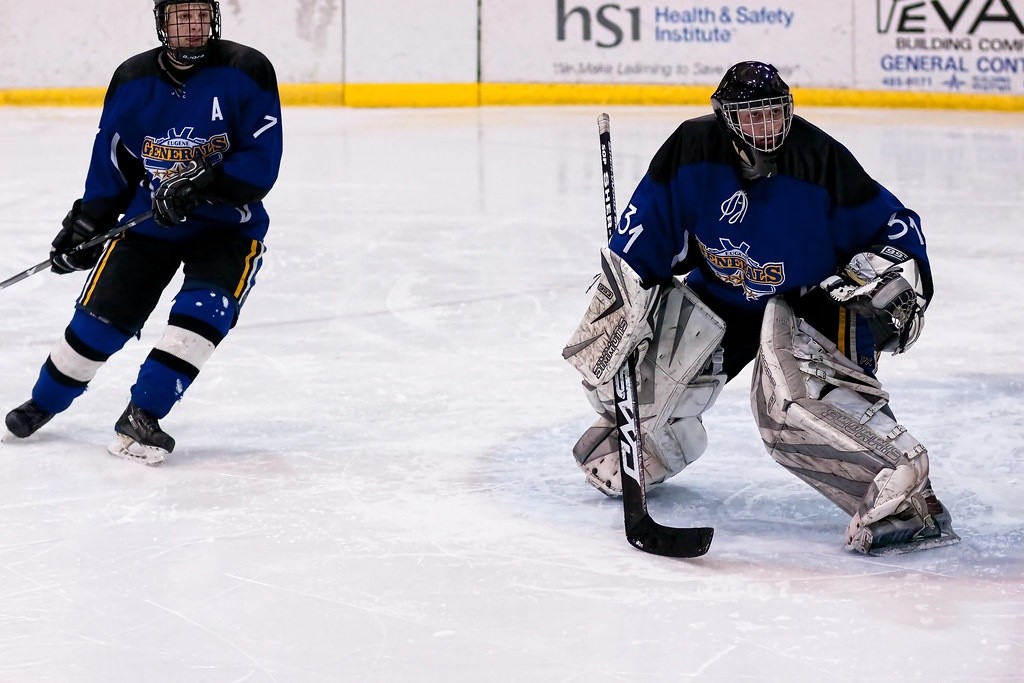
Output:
[1,400,55,442]
[870,477,962,558]
[107,402,175,466]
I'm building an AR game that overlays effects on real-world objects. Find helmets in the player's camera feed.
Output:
[711,61,793,157]
[153,0,222,63]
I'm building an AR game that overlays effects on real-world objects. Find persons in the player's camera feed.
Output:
[0,0,283,465]
[562,62,960,558]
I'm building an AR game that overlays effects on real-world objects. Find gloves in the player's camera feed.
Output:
[50,198,119,274]
[151,161,215,228]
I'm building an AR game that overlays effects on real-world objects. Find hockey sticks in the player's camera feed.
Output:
[596,112,716,561]
[0,211,154,291]
